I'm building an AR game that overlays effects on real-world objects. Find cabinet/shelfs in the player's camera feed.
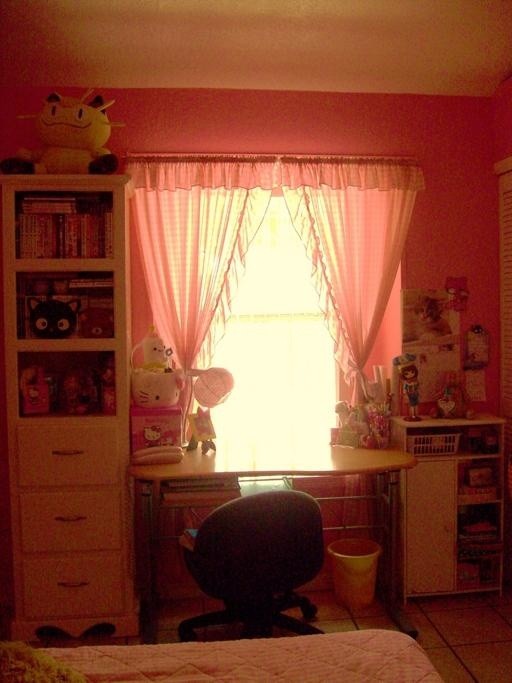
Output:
[392,414,507,611]
[1,168,141,645]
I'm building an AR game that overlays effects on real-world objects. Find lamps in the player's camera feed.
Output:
[181,365,236,449]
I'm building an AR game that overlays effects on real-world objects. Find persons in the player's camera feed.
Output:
[398,360,420,419]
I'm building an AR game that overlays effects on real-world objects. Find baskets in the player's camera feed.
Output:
[407,432,461,457]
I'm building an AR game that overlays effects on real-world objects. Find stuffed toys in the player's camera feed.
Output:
[131,365,185,409]
[141,322,170,370]
[0,86,125,174]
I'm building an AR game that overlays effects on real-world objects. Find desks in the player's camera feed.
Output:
[128,441,414,625]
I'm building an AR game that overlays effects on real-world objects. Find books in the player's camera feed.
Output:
[13,193,114,339]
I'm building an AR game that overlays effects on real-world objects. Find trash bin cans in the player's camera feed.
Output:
[327,537,382,605]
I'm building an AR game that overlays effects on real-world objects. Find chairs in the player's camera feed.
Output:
[176,488,328,640]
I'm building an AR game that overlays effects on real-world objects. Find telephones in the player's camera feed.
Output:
[132,445,184,465]
